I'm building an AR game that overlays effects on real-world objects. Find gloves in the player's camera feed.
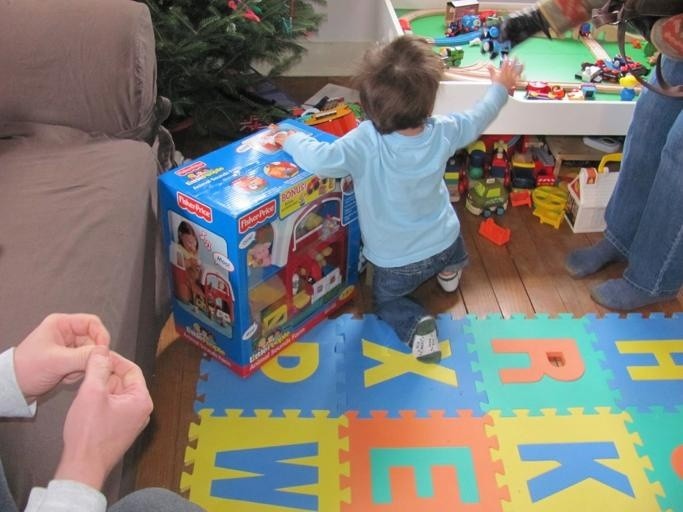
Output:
[629,18,653,44]
[479,7,547,59]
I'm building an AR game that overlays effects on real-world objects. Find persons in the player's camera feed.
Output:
[275,35,524,364]
[480,0,683,310]
[169,221,205,306]
[0,313,209,512]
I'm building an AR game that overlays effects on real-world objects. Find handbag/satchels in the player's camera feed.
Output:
[593,0,683,29]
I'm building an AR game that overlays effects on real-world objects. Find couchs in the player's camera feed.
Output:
[0,0,176,511]
